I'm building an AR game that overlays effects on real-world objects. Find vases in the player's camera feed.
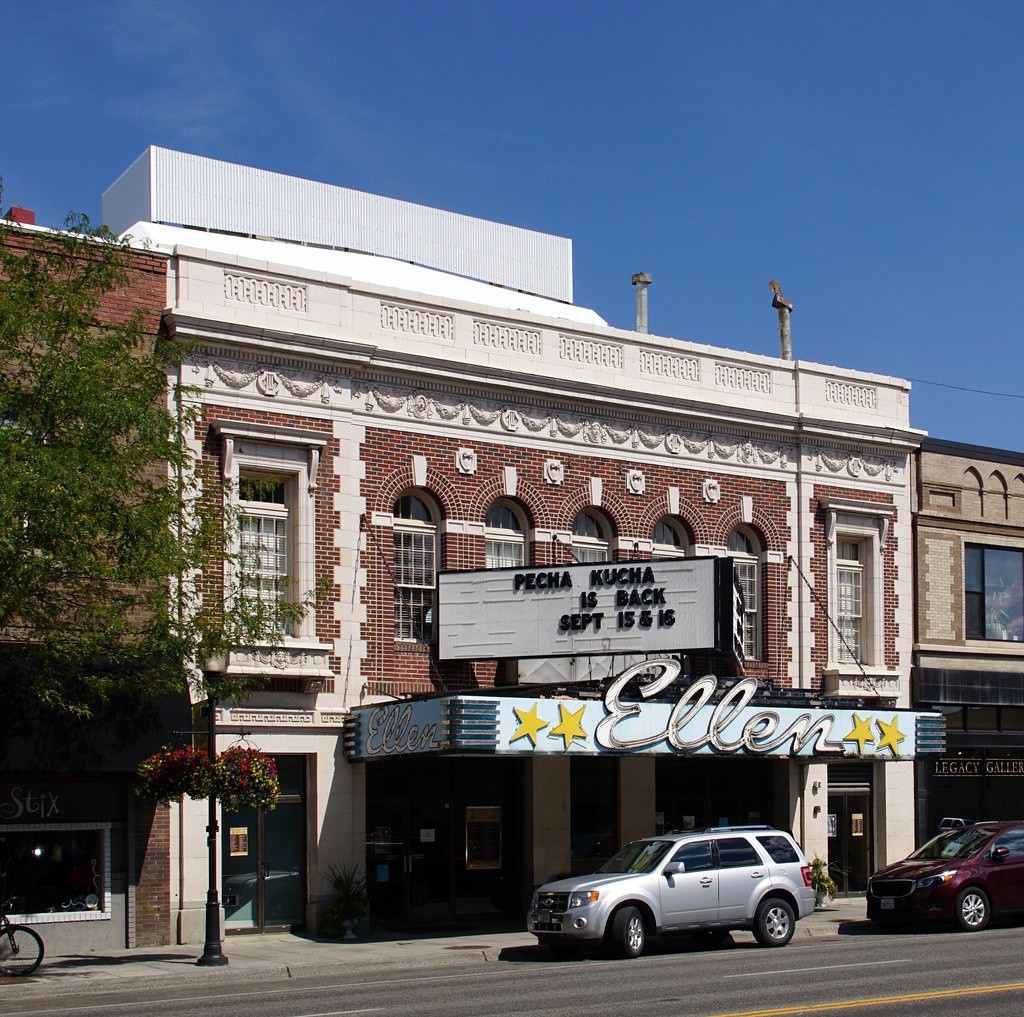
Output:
[815,888,829,908]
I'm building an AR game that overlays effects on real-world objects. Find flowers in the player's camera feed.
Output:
[131,731,214,806]
[806,849,839,902]
[213,737,281,814]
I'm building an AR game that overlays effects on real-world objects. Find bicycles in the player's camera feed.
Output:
[0,894,46,977]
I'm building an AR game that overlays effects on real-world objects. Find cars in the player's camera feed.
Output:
[935,817,973,836]
[866,820,1024,933]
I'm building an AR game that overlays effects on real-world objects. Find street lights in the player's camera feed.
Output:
[197,632,230,967]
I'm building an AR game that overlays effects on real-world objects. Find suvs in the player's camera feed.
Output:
[526,825,818,958]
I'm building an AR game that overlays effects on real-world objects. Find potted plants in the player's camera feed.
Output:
[318,854,374,941]
